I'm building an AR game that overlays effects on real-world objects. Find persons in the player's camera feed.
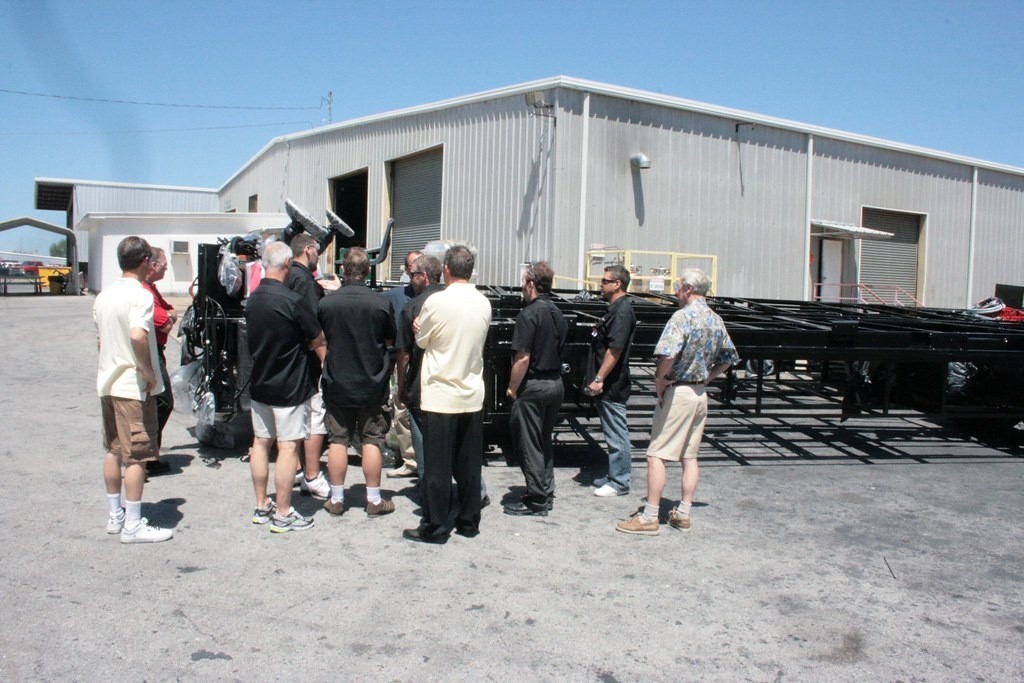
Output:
[586,264,636,497]
[316,246,399,518]
[403,246,492,544]
[504,262,569,516]
[92,236,174,543]
[283,234,332,498]
[316,251,425,478]
[394,255,490,510]
[617,267,740,535]
[140,247,177,478]
[243,242,328,533]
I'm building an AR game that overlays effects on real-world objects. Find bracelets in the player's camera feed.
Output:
[168,311,172,318]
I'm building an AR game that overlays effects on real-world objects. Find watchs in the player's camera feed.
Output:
[595,376,603,383]
[507,390,512,396]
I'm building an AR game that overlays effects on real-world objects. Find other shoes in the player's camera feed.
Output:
[120,464,149,478]
[480,495,490,508]
[146,460,171,474]
[403,529,422,541]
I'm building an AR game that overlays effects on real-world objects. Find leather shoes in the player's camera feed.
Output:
[504,502,548,516]
[503,493,552,510]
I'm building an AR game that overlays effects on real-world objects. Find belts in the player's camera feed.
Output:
[667,381,703,387]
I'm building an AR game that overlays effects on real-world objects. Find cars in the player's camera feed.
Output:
[0,259,62,277]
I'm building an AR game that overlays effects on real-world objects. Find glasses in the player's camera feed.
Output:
[602,278,624,284]
[410,272,429,279]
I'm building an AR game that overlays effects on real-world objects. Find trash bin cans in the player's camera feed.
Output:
[49,276,64,295]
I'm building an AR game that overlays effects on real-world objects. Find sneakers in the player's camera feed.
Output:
[663,510,691,533]
[322,498,345,517]
[252,498,277,523]
[107,507,125,534]
[615,512,660,536]
[300,471,330,497]
[269,507,315,532]
[594,474,608,487]
[293,471,304,486]
[595,482,629,497]
[386,465,418,477]
[120,517,173,543]
[367,498,395,518]
